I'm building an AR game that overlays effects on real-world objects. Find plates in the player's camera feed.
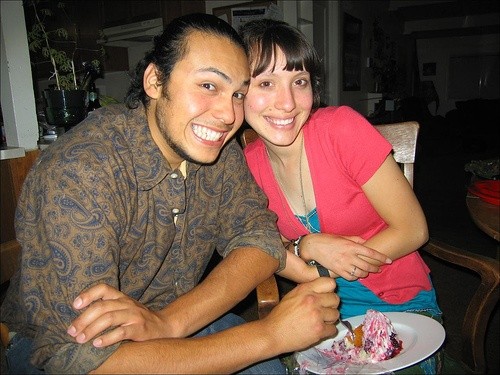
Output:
[469,179,500,204]
[293,311,448,375]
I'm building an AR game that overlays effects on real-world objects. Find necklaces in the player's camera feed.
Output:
[264,134,310,233]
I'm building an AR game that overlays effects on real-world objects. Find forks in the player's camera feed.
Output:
[312,256,356,341]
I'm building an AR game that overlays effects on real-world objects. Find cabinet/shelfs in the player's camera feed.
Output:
[104,0,162,28]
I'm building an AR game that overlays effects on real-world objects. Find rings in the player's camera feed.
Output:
[349,267,356,275]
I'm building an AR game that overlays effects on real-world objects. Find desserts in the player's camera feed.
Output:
[333,309,396,363]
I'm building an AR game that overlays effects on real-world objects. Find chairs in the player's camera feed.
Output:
[241,121,500,375]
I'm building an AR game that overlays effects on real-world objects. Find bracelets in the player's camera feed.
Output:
[316,264,330,277]
[293,234,316,266]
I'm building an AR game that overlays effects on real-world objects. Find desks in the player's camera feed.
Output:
[465,189,500,244]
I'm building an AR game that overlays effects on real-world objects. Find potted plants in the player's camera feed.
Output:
[25,0,108,128]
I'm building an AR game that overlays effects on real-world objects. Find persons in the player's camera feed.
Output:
[237,19,443,374]
[0,15,339,374]
